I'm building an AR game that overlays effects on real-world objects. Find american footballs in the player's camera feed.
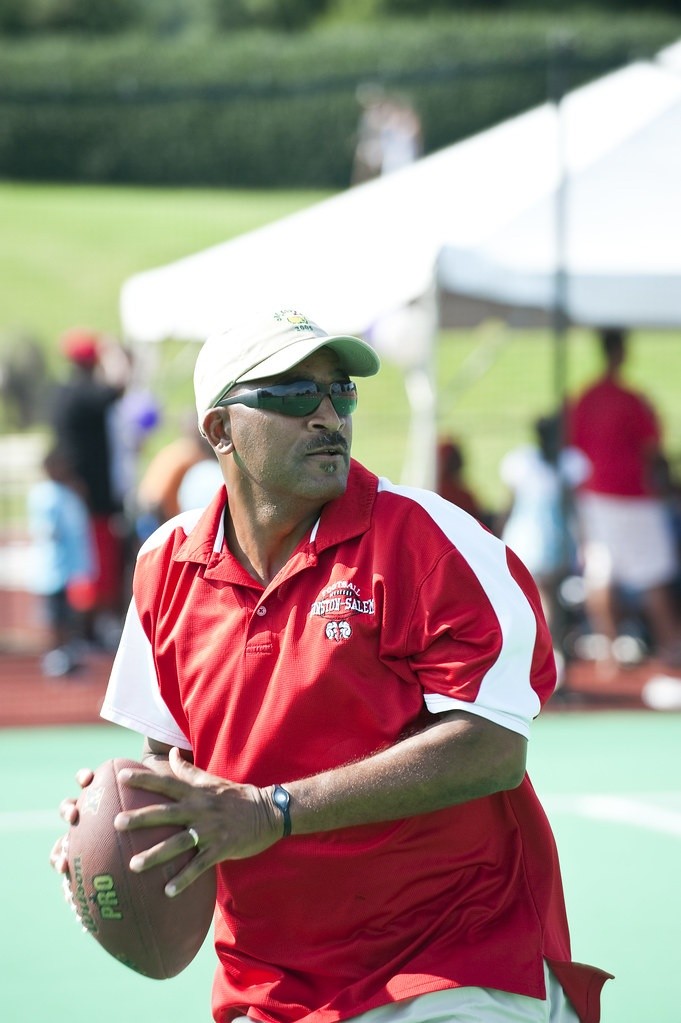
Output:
[65,759,219,981]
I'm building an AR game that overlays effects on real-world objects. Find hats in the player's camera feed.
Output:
[195,307,379,441]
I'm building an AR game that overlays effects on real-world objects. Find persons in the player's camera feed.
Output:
[0,329,681,678]
[46,302,615,1022]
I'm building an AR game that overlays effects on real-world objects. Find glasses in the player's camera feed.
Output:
[216,379,357,417]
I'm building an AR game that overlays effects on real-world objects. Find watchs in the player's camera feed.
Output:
[270,782,293,838]
